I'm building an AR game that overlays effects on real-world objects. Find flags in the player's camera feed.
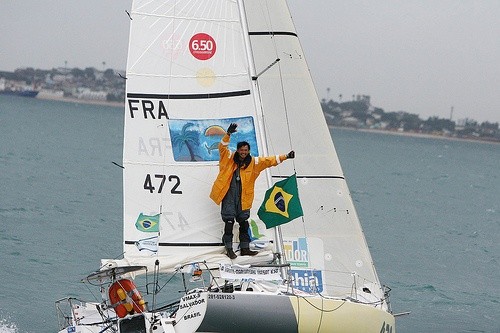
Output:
[135,213,158,232]
[256,174,304,230]
[136,236,159,253]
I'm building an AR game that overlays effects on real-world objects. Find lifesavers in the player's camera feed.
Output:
[109,279,144,318]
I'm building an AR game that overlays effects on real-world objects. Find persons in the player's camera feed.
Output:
[209,122,294,259]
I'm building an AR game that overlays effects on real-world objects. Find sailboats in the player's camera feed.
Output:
[54,0,413,333]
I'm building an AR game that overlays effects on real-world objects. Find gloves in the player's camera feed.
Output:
[286,151,294,159]
[227,123,237,135]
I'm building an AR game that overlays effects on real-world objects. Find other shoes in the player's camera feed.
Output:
[227,248,237,259]
[241,248,258,256]
